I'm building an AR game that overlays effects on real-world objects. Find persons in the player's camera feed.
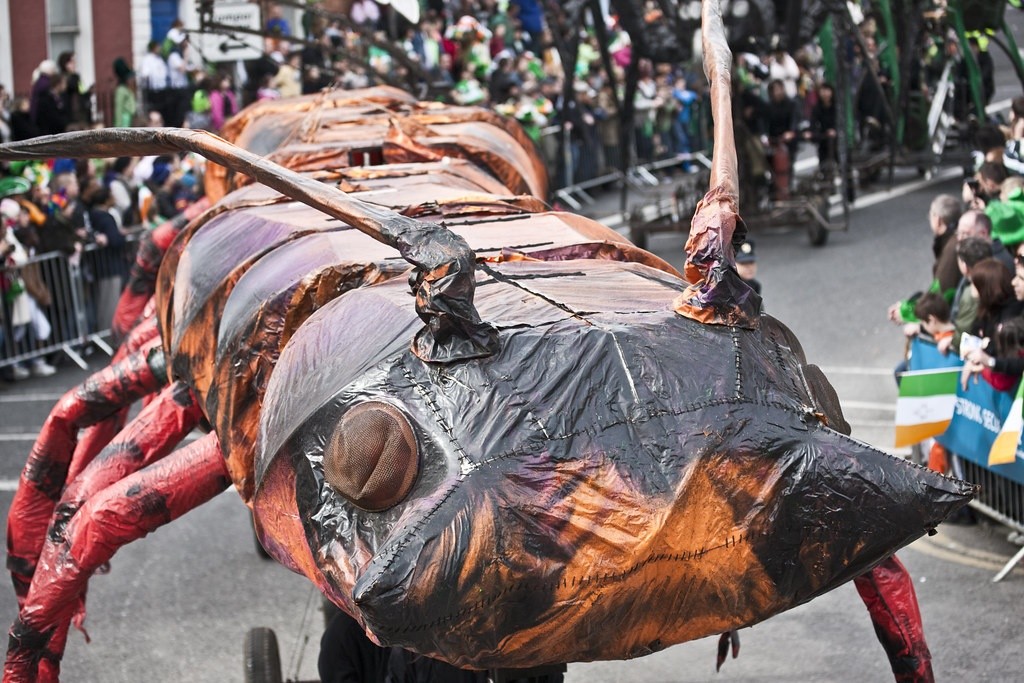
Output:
[888,96,1024,391]
[0,0,993,378]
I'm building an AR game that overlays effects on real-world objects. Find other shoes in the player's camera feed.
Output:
[32,359,58,377]
[13,365,32,381]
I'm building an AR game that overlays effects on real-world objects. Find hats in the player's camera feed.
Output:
[573,81,595,97]
[986,189,1024,243]
